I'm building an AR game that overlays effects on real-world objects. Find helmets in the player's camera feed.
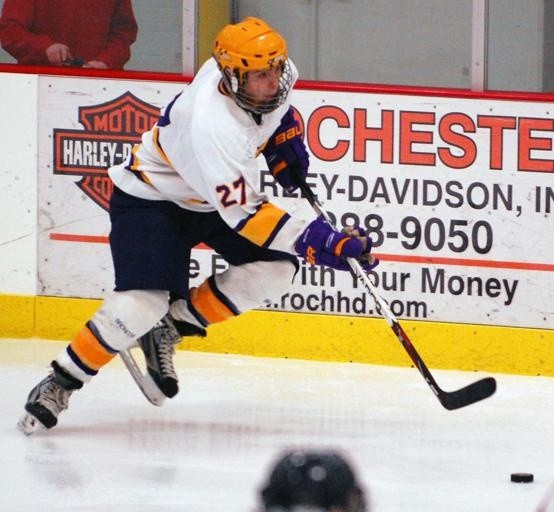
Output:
[213,15,296,114]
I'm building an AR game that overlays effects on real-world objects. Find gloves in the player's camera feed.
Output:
[294,215,379,275]
[259,116,309,191]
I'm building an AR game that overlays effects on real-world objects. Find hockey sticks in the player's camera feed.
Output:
[290,166,495,411]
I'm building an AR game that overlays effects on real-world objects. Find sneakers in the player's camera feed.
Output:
[26,369,68,428]
[135,297,180,400]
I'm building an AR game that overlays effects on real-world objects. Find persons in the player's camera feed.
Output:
[26,16,380,430]
[1,0,138,71]
